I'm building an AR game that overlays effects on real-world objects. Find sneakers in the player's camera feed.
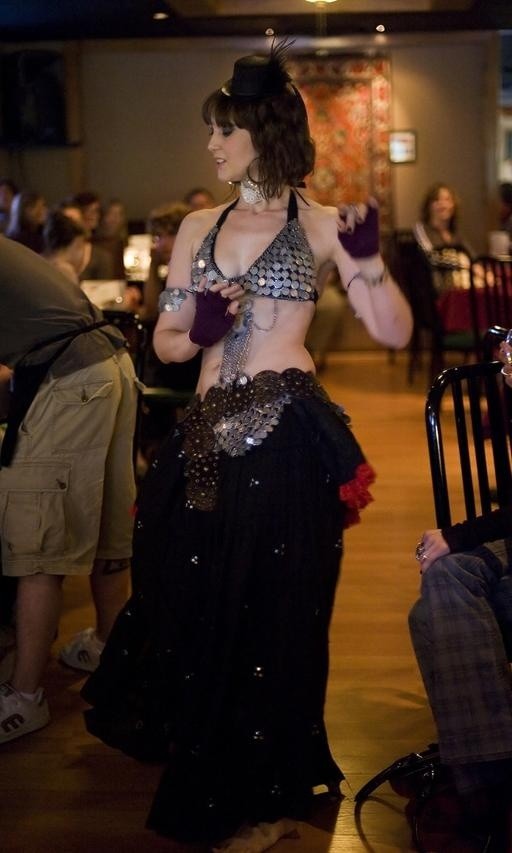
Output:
[59,627,107,672]
[0,681,51,744]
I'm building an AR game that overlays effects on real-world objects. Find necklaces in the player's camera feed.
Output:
[236,179,290,208]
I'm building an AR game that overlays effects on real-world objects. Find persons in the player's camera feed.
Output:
[401,177,494,289]
[76,32,421,852]
[407,323,512,852]
[0,175,219,467]
[1,232,136,744]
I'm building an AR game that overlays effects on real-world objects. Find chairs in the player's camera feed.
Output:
[99,306,192,463]
[383,221,512,387]
[424,358,511,560]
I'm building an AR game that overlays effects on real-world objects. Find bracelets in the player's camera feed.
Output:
[354,263,394,289]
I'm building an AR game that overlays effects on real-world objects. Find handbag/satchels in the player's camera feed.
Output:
[354,741,439,829]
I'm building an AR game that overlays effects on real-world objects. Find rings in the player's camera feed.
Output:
[413,542,426,561]
